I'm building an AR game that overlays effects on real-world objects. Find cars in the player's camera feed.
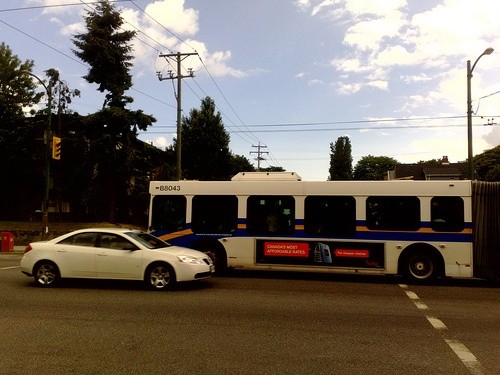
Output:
[20,228,216,291]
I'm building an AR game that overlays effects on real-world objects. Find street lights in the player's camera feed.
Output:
[145,170,500,287]
[13,67,64,233]
[466,48,494,177]
